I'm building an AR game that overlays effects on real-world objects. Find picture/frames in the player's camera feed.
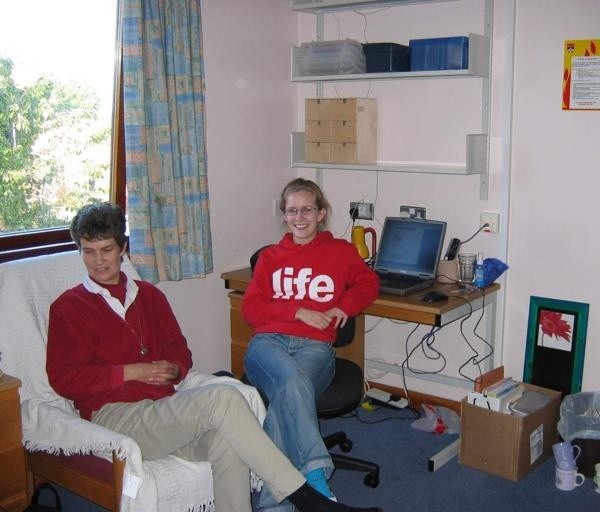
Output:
[523,294,591,402]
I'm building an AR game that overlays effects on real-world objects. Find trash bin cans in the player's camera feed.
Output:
[556,391,600,478]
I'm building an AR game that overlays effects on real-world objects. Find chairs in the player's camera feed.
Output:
[241,244,382,490]
[0,249,268,512]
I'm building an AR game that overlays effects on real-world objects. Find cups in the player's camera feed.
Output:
[554,464,585,491]
[457,252,478,283]
[594,463,600,494]
[551,441,581,471]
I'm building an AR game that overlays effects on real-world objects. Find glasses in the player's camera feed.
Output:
[286,207,319,217]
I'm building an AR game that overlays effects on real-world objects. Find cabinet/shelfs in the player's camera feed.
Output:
[289,1,495,201]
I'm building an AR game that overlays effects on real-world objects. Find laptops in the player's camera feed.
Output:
[372,216,447,297]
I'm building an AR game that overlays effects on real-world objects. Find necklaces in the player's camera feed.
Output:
[96,300,151,356]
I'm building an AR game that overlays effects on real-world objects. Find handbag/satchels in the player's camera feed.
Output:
[26,483,61,512]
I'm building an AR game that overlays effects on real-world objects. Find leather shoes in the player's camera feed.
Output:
[297,504,383,511]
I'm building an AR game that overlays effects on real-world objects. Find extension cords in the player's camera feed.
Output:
[366,387,409,409]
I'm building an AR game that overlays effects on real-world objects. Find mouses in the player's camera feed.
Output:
[423,290,449,304]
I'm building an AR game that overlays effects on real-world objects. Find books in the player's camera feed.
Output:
[467,366,524,412]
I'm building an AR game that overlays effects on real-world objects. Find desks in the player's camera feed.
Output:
[0,372,30,512]
[218,266,501,473]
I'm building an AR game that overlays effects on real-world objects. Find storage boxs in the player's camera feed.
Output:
[363,43,409,73]
[457,376,564,482]
[409,34,471,72]
[306,98,378,165]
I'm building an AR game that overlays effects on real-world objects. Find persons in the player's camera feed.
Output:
[239,177,381,512]
[45,201,384,512]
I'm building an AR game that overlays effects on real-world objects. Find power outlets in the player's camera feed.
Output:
[273,198,283,217]
[479,212,501,235]
